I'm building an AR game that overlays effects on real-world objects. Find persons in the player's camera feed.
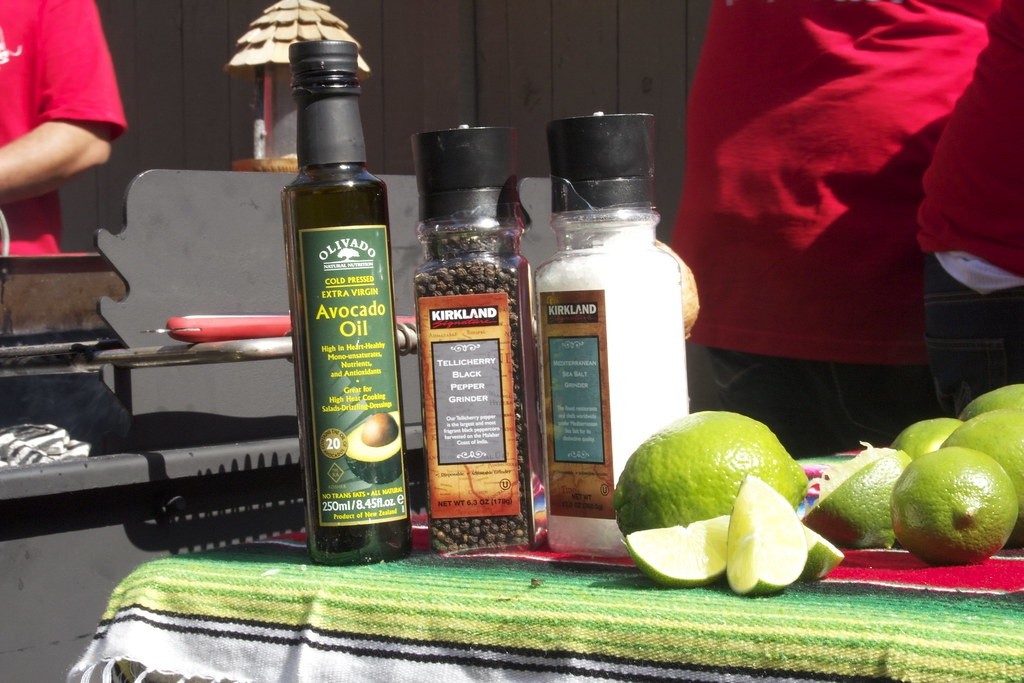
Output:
[670,0,1007,457]
[913,1,1024,417]
[0,0,129,256]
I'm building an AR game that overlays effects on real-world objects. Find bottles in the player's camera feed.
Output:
[279,40,412,565]
[532,113,691,558]
[411,126,546,555]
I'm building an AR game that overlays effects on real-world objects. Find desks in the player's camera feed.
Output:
[68,440,1023,683]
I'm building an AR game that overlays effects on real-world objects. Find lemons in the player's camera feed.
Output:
[611,381,1024,596]
